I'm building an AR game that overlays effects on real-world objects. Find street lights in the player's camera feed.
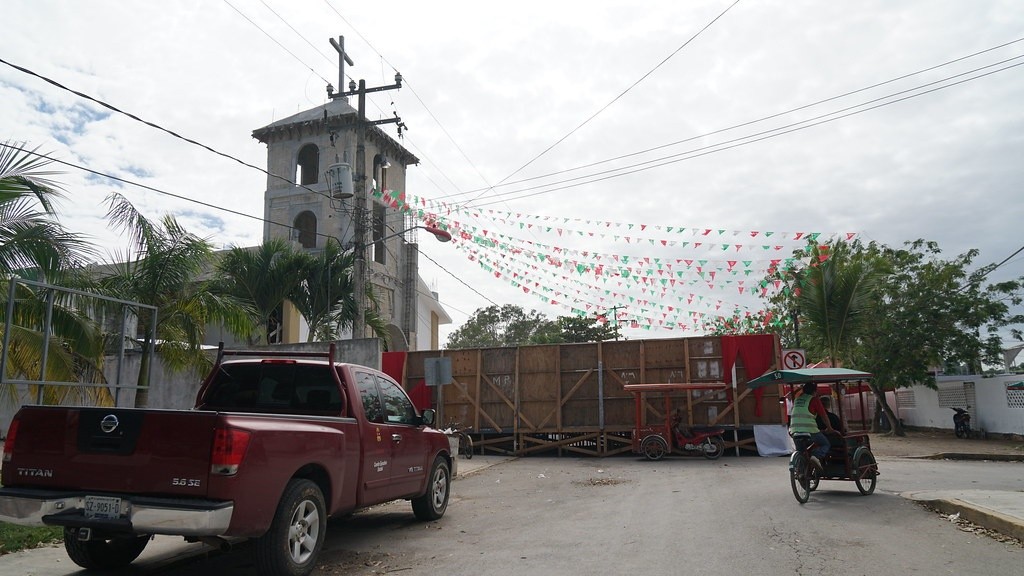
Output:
[354,224,454,338]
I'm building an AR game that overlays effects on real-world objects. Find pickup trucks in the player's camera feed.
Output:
[0,342,462,576]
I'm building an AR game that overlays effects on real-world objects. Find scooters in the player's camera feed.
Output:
[951,404,972,437]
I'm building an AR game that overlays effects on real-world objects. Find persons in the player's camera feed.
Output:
[790,381,845,474]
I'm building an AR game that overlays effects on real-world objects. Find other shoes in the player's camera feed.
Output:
[810,455,823,473]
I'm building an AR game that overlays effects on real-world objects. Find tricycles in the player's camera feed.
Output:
[747,363,880,503]
[626,380,731,459]
